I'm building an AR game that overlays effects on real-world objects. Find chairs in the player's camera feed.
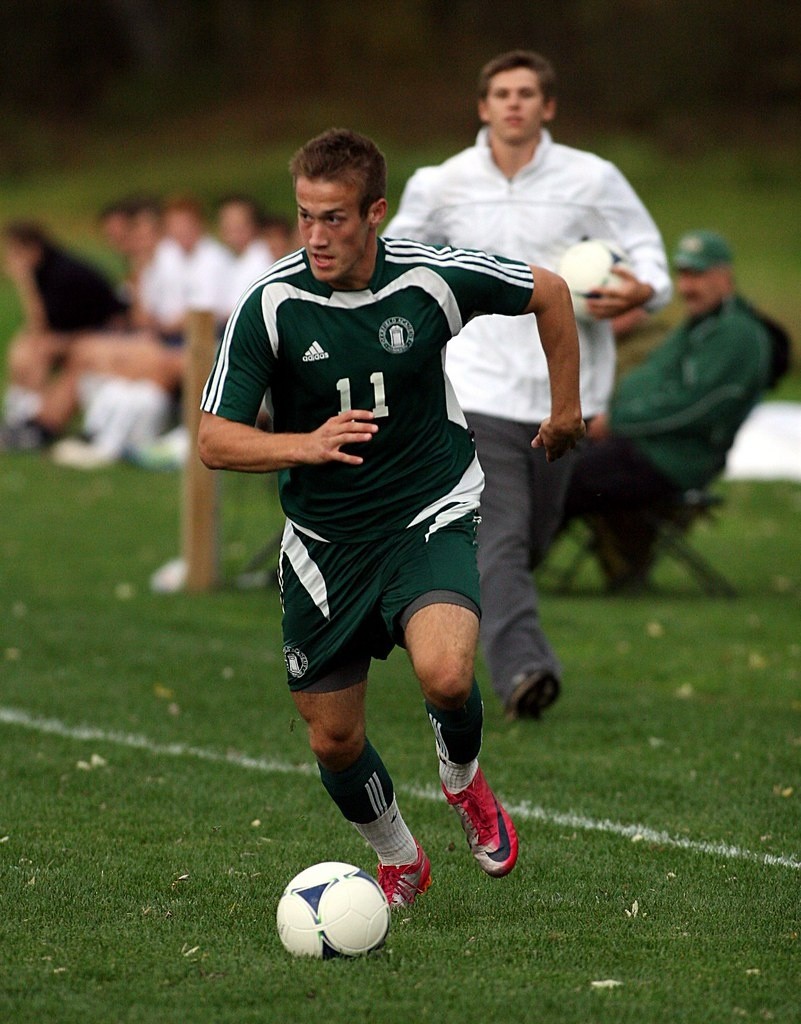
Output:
[539,318,791,603]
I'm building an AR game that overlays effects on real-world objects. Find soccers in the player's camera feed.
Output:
[277,862,391,959]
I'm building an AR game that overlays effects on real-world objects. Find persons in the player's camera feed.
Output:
[560,233,773,541]
[385,49,674,724]
[195,131,586,907]
[2,181,307,474]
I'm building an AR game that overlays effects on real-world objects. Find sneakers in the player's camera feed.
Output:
[376,840,430,920]
[497,659,563,725]
[440,766,520,876]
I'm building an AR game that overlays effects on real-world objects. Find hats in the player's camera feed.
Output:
[674,230,727,272]
